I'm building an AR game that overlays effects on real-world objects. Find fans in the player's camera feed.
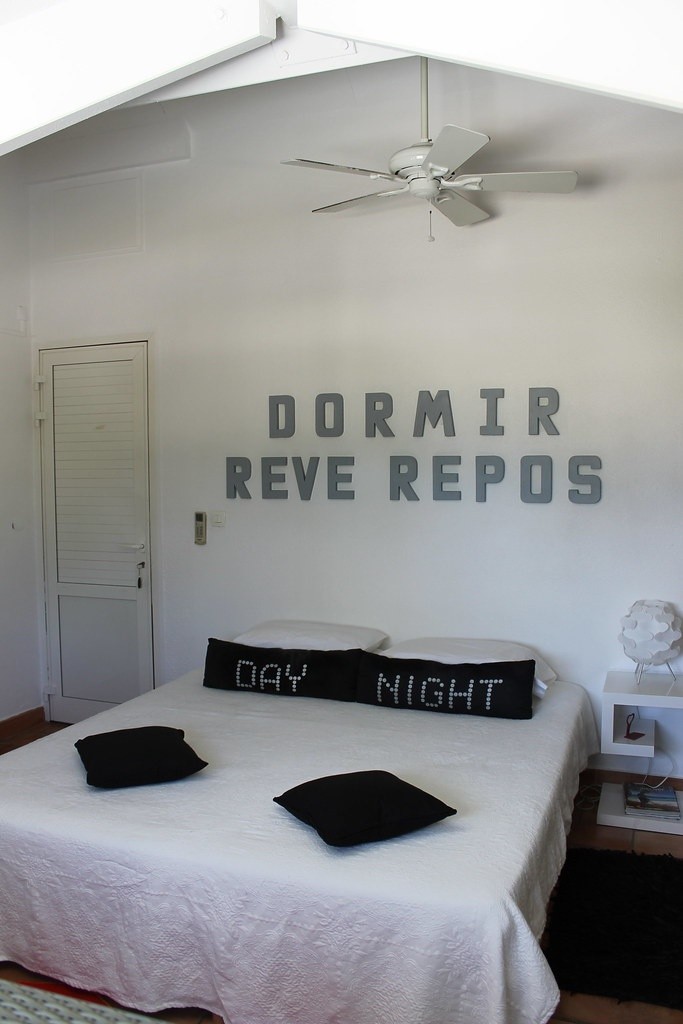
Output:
[282,57,578,227]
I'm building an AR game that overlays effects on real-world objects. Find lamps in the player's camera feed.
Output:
[617,599,683,684]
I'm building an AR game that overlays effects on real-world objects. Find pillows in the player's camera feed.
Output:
[379,637,557,699]
[354,652,537,720]
[233,621,388,656]
[75,726,209,789]
[202,638,362,702]
[273,770,457,846]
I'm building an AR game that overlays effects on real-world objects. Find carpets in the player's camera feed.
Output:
[546,846,683,1011]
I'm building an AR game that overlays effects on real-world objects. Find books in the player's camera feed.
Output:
[623,780,680,820]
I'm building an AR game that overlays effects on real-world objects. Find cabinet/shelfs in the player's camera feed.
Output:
[596,671,683,837]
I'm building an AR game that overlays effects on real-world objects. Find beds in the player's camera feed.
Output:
[0,668,600,1024]
